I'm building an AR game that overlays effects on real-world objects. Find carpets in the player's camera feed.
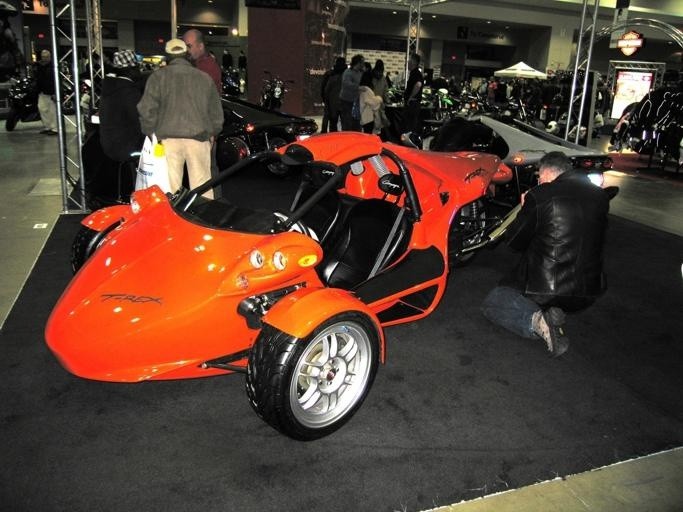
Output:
[2,200,681,512]
[61,171,111,212]
[596,149,683,191]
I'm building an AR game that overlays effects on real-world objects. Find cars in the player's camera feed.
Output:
[141,55,169,68]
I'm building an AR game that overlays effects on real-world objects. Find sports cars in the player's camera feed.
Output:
[209,97,317,162]
[395,114,615,216]
[43,116,621,440]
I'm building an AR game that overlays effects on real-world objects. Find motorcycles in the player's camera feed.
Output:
[4,65,41,131]
[257,69,293,113]
[59,73,92,114]
[220,67,241,100]
[382,85,535,137]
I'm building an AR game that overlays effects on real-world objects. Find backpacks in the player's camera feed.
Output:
[349,90,367,121]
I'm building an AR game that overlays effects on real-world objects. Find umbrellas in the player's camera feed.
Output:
[493,60,548,81]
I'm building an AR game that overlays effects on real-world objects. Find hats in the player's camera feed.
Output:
[163,38,187,55]
[108,49,141,70]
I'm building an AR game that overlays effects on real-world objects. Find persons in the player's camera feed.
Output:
[99,49,153,160]
[479,149,611,360]
[182,29,222,200]
[320,52,514,133]
[134,37,226,200]
[36,49,60,136]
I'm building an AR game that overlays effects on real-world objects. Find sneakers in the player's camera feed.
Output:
[47,132,58,135]
[533,305,570,359]
[39,130,50,134]
